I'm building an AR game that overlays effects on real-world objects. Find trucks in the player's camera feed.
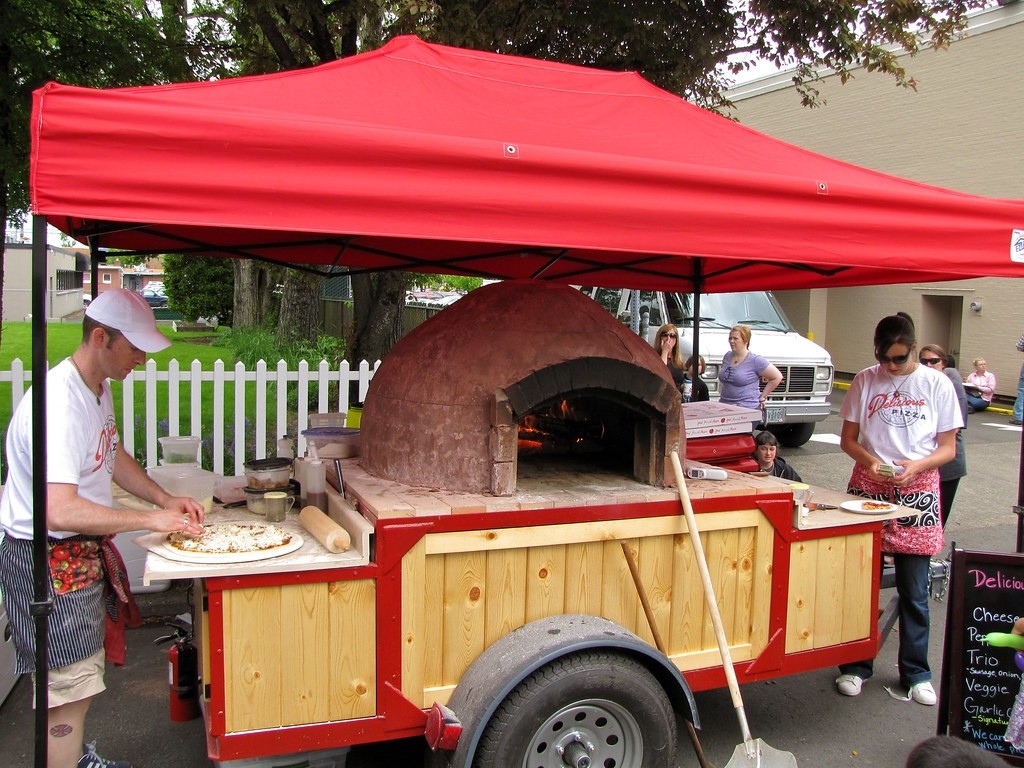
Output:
[567,284,835,447]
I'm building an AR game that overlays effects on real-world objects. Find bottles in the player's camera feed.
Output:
[276,437,330,515]
[347,402,363,429]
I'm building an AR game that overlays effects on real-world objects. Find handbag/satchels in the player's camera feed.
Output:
[964,386,983,398]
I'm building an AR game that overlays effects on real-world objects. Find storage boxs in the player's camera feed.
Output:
[147,466,222,513]
[110,530,171,593]
[682,401,762,438]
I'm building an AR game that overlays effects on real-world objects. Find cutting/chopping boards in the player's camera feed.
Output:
[130,521,304,565]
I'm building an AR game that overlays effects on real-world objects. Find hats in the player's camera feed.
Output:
[84,286,172,354]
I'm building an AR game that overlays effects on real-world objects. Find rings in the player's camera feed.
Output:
[183,518,188,524]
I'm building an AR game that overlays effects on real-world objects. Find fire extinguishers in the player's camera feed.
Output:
[155,622,199,723]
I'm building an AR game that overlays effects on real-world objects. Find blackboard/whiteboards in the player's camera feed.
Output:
[936,548,1024,768]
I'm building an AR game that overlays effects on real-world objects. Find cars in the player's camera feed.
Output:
[138,289,169,307]
[83,294,92,307]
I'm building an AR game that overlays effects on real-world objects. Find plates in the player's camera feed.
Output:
[840,499,899,514]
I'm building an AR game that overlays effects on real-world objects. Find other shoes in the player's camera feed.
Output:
[968,406,974,414]
[1009,418,1022,425]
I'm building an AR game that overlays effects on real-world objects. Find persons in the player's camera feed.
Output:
[919,345,968,532]
[1009,333,1024,424]
[754,431,804,484]
[963,355,995,414]
[907,736,1011,768]
[678,354,710,403]
[1003,618,1024,752]
[835,310,965,705]
[718,325,783,432]
[1,286,206,768]
[653,324,684,398]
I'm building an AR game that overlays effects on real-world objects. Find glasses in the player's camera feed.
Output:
[919,357,944,366]
[659,331,677,339]
[875,345,912,364]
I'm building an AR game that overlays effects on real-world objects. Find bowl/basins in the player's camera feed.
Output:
[243,458,294,514]
[157,436,201,463]
[302,427,360,460]
[308,414,346,429]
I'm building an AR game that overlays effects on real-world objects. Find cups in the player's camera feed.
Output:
[264,491,295,521]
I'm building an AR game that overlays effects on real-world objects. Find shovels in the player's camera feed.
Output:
[670,451,797,768]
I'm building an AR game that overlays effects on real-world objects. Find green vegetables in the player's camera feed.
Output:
[165,453,197,463]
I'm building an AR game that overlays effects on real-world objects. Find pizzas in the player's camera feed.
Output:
[160,523,292,557]
[862,502,891,511]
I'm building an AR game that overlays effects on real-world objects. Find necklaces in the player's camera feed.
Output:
[887,363,915,398]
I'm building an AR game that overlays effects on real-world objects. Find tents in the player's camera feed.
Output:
[31,34,1024,768]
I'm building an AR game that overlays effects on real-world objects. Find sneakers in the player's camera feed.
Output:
[836,674,863,696]
[76,740,132,768]
[883,679,937,705]
